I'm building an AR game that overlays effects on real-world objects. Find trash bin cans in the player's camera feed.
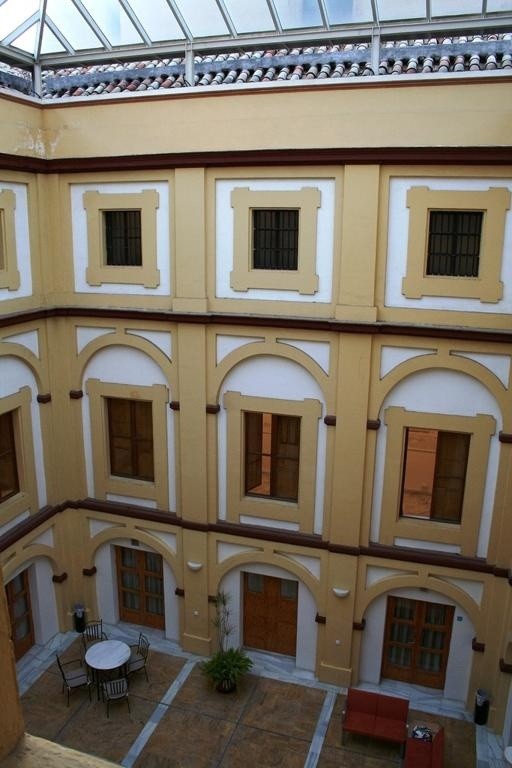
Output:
[73,604,86,632]
[473,687,492,726]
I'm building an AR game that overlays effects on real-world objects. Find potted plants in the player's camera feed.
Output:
[196,587,254,699]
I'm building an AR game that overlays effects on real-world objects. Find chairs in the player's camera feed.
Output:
[55,619,151,720]
[341,685,445,768]
